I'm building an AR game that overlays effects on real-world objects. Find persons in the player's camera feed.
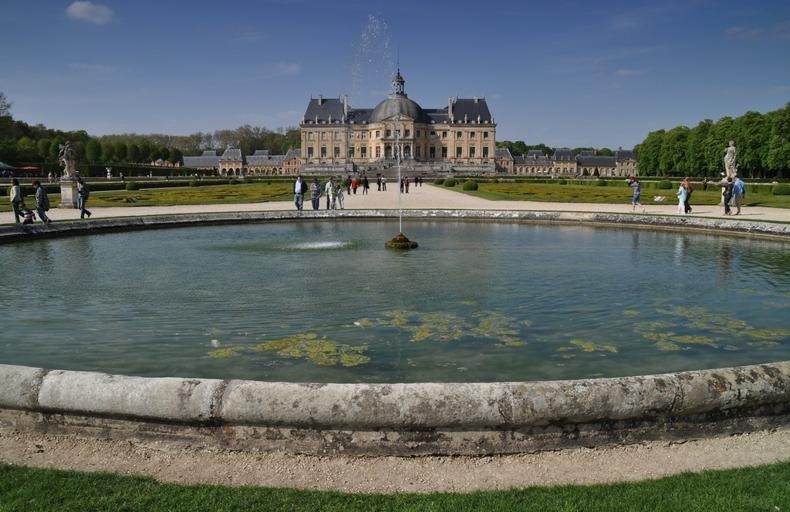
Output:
[724,140,738,177]
[292,155,423,211]
[8,170,92,224]
[59,140,75,178]
[120,172,125,185]
[674,176,745,216]
[628,176,645,213]
